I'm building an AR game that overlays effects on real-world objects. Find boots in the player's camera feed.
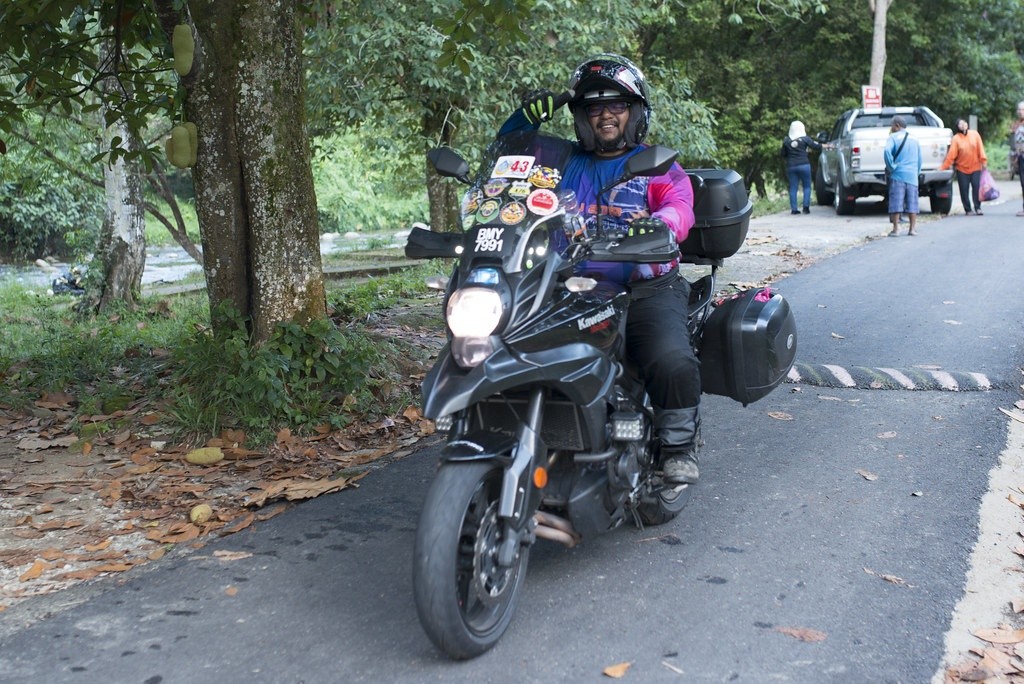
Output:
[650,403,703,484]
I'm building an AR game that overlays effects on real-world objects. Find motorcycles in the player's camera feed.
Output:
[404,146,799,662]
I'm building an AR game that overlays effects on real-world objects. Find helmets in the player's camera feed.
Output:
[567,52,652,149]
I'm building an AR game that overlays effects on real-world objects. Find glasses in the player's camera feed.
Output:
[586,102,631,116]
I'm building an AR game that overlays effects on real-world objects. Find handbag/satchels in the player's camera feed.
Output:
[978,170,1000,202]
[884,168,891,186]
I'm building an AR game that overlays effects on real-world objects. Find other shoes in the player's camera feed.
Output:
[803,206,810,213]
[966,209,983,215]
[888,231,899,237]
[791,210,801,214]
[908,231,918,235]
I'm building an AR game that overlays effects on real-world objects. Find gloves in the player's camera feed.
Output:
[520,86,576,129]
[627,217,664,237]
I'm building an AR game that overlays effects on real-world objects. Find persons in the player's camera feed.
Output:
[938,118,987,216]
[496,54,701,484]
[884,115,922,236]
[1013,101,1024,216]
[781,121,835,215]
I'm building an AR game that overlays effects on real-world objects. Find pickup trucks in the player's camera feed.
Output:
[812,106,957,217]
